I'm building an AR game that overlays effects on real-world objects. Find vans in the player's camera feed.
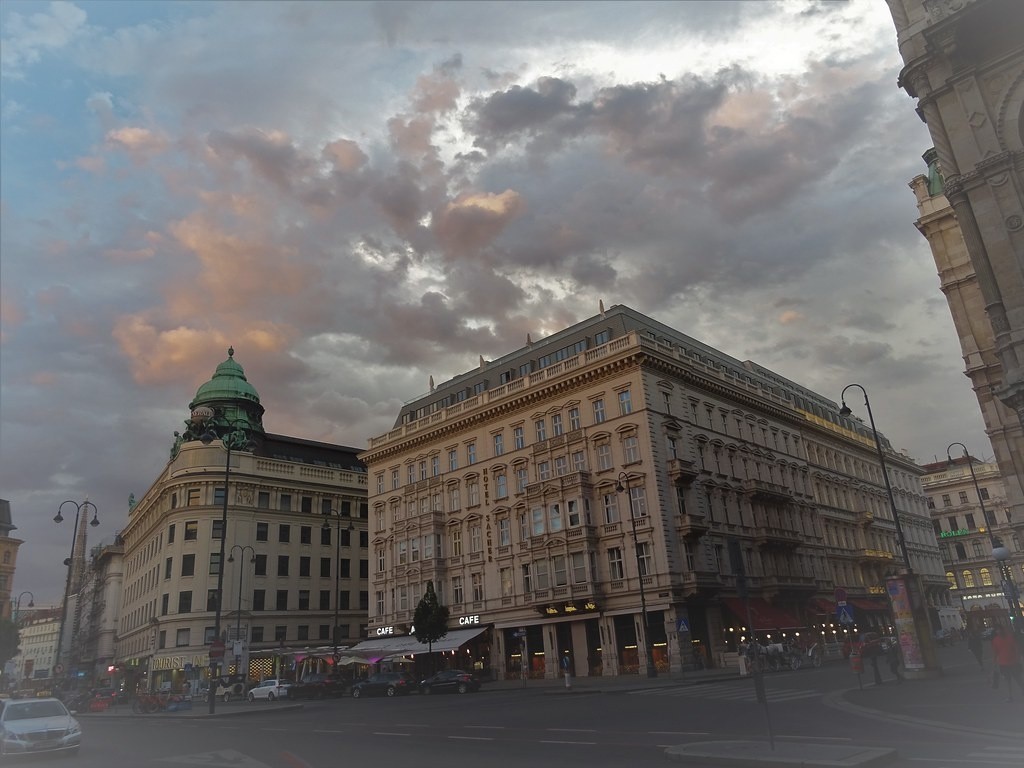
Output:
[844,631,879,655]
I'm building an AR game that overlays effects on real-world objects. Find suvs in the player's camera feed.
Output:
[204,673,247,702]
[350,672,409,696]
[298,672,347,690]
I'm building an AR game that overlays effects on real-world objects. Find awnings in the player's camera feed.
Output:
[347,627,489,654]
[725,600,892,632]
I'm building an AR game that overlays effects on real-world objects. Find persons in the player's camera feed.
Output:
[694,643,708,672]
[739,611,1024,675]
[560,653,574,688]
[993,624,1024,704]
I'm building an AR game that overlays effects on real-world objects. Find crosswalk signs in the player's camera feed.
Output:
[839,608,854,624]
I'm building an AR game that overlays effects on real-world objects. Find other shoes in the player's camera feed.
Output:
[1006,697,1012,702]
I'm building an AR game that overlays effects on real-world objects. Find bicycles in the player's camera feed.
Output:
[130,687,192,714]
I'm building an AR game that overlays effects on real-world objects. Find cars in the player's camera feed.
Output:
[0,697,82,758]
[5,679,129,712]
[880,637,897,650]
[247,679,297,702]
[419,669,482,695]
[934,626,994,644]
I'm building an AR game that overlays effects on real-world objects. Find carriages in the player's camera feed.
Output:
[738,634,823,672]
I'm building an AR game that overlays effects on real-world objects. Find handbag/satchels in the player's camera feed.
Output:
[991,670,999,689]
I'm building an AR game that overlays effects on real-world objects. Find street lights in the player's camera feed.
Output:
[52,500,101,672]
[227,543,258,673]
[945,441,1024,631]
[199,411,257,711]
[617,472,659,678]
[322,507,342,654]
[14,591,34,625]
[838,383,931,650]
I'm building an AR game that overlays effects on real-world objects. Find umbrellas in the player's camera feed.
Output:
[337,656,376,678]
[382,656,415,671]
